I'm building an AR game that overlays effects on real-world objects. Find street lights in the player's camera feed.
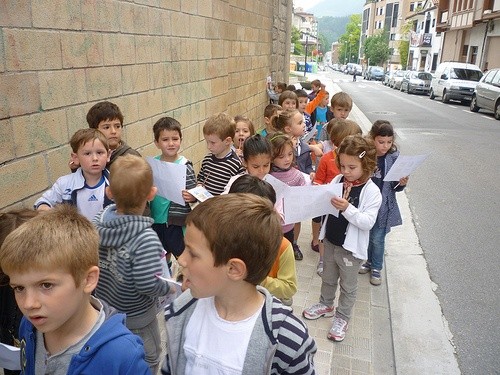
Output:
[304,32,310,76]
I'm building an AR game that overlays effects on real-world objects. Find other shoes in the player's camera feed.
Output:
[312,240,319,252]
[293,243,303,259]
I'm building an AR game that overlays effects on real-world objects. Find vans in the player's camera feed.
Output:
[429,62,485,107]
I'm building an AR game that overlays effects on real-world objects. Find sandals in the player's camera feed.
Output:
[317,260,323,276]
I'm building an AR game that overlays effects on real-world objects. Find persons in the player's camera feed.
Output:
[158,192,318,375]
[0,79,410,375]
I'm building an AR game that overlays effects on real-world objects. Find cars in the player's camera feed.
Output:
[382,70,397,86]
[328,62,386,81]
[470,68,500,121]
[399,70,433,96]
[389,70,412,90]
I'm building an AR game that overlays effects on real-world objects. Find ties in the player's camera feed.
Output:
[343,174,368,201]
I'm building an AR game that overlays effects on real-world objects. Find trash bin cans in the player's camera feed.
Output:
[306,62,318,72]
[290,60,296,70]
[297,62,305,71]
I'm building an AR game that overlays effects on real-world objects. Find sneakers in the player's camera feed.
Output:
[303,301,335,320]
[358,260,371,274]
[370,266,381,285]
[327,316,349,342]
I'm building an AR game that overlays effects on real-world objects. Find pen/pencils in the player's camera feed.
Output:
[239,138,242,149]
[282,198,285,213]
[202,172,210,187]
[393,175,410,190]
[154,274,182,287]
